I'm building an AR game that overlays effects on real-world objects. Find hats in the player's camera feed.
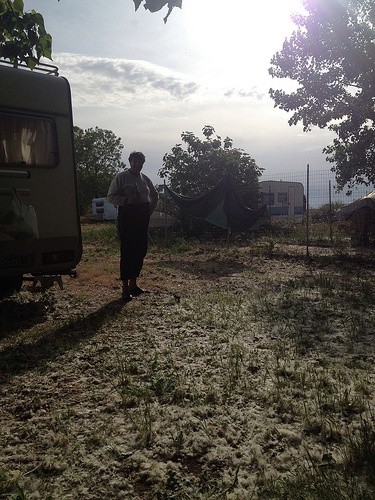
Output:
[129,152,145,163]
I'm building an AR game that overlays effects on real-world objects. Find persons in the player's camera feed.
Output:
[107,152,160,301]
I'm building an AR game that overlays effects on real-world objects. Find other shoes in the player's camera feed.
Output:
[122,290,132,300]
[129,287,146,293]
